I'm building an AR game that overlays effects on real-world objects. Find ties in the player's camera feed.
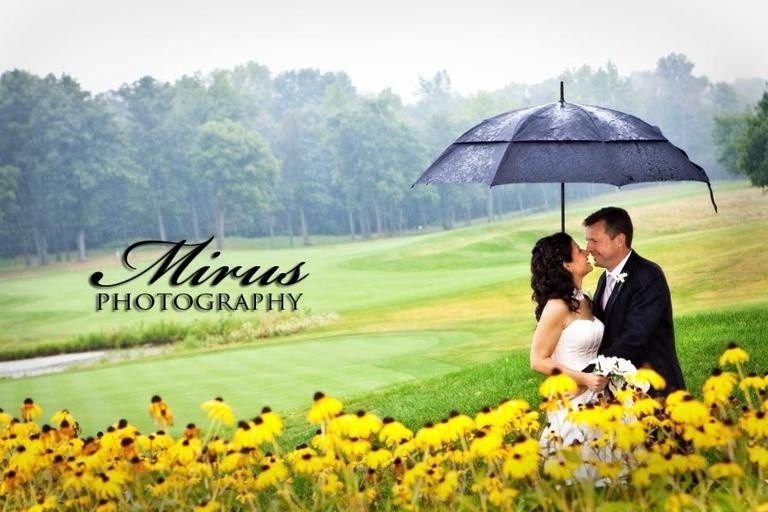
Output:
[602,275,615,312]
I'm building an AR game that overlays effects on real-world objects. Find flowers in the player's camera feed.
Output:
[584,355,650,406]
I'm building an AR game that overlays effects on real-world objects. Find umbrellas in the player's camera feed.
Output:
[410,81,709,232]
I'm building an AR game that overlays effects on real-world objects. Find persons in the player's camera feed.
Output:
[529,207,687,487]
